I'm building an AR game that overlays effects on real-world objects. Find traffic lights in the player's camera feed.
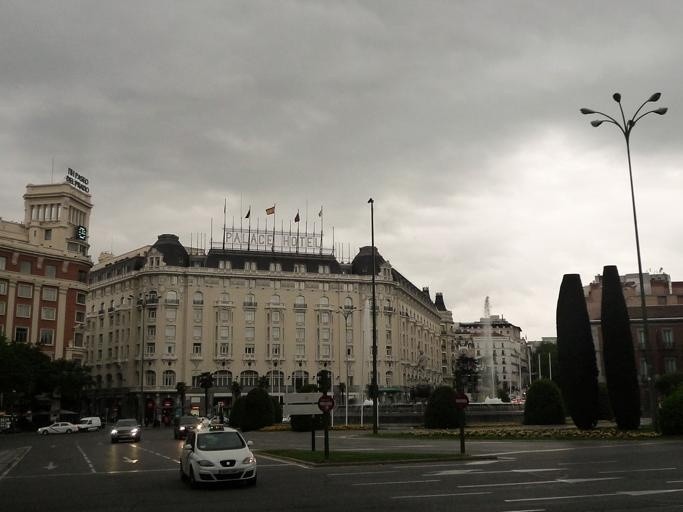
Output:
[198,372,205,388]
[205,372,213,387]
[174,381,183,396]
[316,369,329,392]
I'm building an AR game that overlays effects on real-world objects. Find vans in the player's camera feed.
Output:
[76,416,100,432]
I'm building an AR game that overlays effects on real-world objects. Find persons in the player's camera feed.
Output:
[22,407,33,432]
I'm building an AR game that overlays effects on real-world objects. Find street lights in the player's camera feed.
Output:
[365,196,379,435]
[579,90,670,430]
[126,292,161,426]
[331,303,363,427]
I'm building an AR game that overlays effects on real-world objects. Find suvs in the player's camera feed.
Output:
[109,418,141,442]
[173,415,198,440]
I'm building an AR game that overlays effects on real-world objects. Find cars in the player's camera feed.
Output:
[0,415,37,433]
[177,422,257,486]
[282,415,290,424]
[36,422,79,435]
[510,393,527,404]
[196,416,229,429]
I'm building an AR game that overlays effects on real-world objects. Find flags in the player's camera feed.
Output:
[265,207,274,215]
[295,213,300,223]
[319,210,321,217]
[245,210,250,218]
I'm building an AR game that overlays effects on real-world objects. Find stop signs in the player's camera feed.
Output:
[316,395,335,412]
[452,392,468,409]
